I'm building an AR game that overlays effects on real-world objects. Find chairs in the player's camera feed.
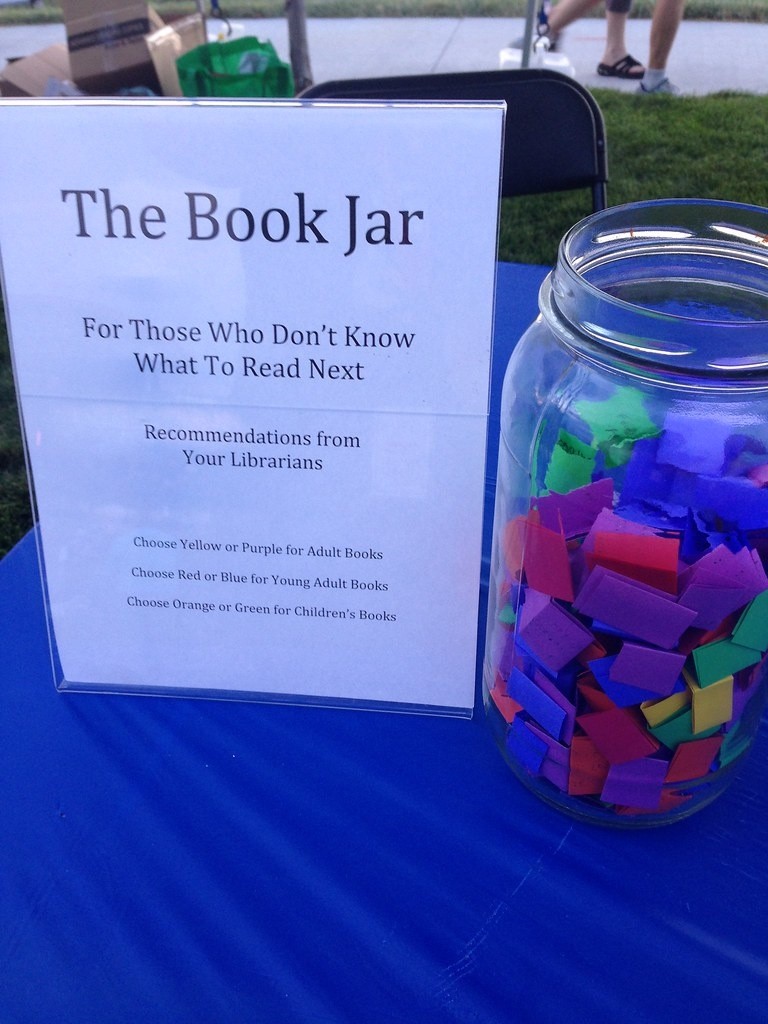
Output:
[294,61,616,227]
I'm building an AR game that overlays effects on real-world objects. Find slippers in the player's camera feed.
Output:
[598,56,646,79]
[539,11,558,54]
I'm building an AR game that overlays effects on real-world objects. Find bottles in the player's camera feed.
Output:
[476,194,767,834]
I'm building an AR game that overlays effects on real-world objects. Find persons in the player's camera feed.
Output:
[524,0,648,79]
[636,0,707,99]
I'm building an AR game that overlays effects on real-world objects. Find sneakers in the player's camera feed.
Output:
[635,77,680,97]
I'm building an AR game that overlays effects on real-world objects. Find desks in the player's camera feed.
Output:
[3,239,768,1024]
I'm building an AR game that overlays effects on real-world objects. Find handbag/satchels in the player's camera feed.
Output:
[176,37,297,98]
